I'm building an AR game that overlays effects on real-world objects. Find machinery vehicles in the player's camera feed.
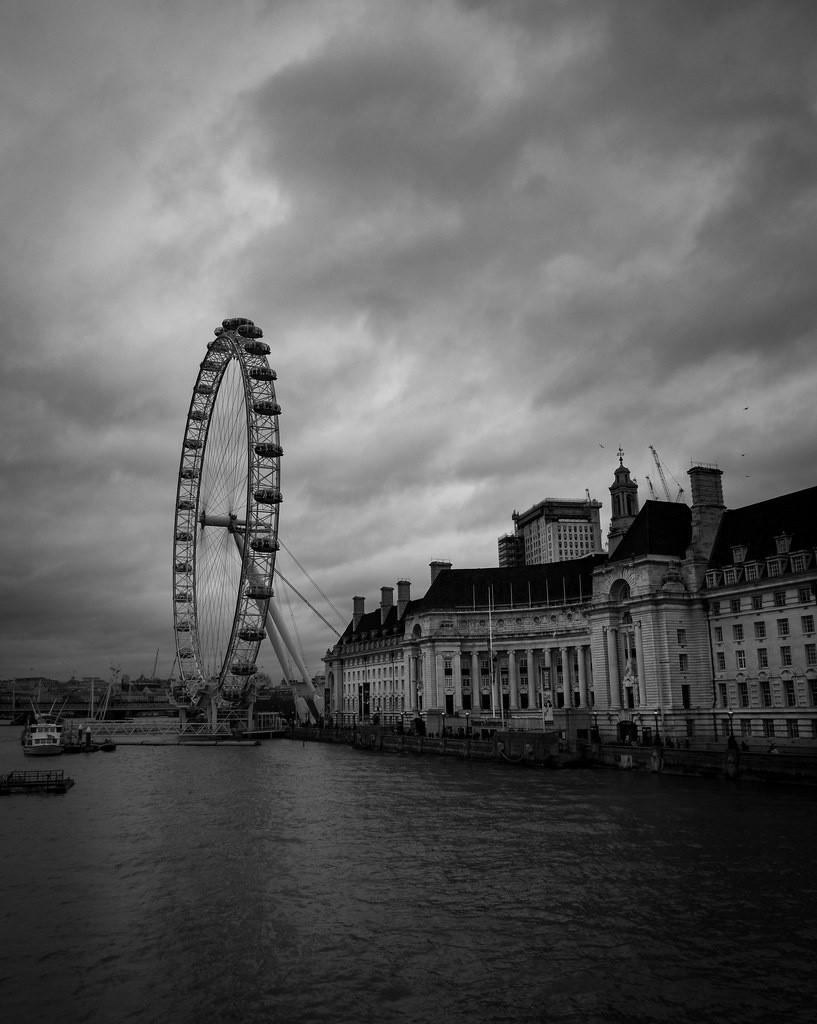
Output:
[645,445,684,503]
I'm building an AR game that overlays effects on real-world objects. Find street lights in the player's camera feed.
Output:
[292,712,295,725]
[401,712,405,726]
[353,711,356,728]
[319,712,322,721]
[653,709,661,743]
[728,708,734,735]
[373,711,376,725]
[335,711,338,728]
[465,711,469,738]
[441,711,446,737]
[593,710,601,742]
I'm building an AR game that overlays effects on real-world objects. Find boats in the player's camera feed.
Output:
[21,714,66,756]
